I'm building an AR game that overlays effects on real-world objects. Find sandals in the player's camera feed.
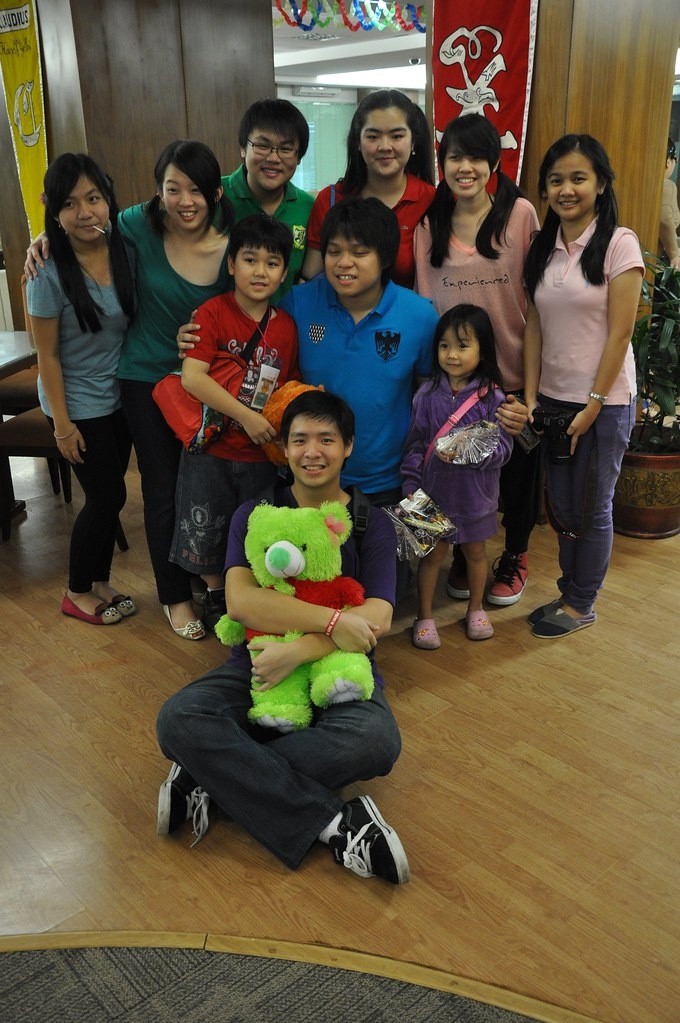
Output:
[192,590,206,606]
[163,604,206,640]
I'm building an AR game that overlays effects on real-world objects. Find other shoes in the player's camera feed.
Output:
[527,598,564,626]
[531,607,597,637]
[201,588,227,631]
[60,589,137,624]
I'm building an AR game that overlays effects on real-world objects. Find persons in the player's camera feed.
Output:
[177,197,529,507]
[25,153,140,625]
[400,303,515,566]
[156,390,445,885]
[171,214,301,637]
[25,139,235,640]
[413,113,540,649]
[219,97,316,307]
[302,89,442,294]
[519,134,646,639]
[654,138,680,303]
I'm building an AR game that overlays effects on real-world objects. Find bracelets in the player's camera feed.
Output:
[54,423,77,439]
[324,609,342,637]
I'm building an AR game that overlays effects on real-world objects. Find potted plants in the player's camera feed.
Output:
[611,240,680,541]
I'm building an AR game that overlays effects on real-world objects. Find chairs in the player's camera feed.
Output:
[0,274,61,495]
[0,404,130,552]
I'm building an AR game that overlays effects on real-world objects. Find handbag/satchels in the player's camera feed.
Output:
[152,349,248,455]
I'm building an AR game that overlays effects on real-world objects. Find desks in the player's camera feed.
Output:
[0,330,38,381]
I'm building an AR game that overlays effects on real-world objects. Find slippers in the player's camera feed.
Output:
[465,609,494,640]
[412,616,439,649]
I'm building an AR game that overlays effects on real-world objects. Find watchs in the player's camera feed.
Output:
[588,392,608,405]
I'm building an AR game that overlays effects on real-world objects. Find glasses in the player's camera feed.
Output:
[247,138,299,159]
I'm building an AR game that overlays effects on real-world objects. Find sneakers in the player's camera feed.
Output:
[329,794,411,884]
[156,762,209,847]
[447,543,469,598]
[487,551,528,605]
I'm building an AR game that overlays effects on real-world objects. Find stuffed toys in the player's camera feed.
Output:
[260,380,324,469]
[214,502,375,733]
